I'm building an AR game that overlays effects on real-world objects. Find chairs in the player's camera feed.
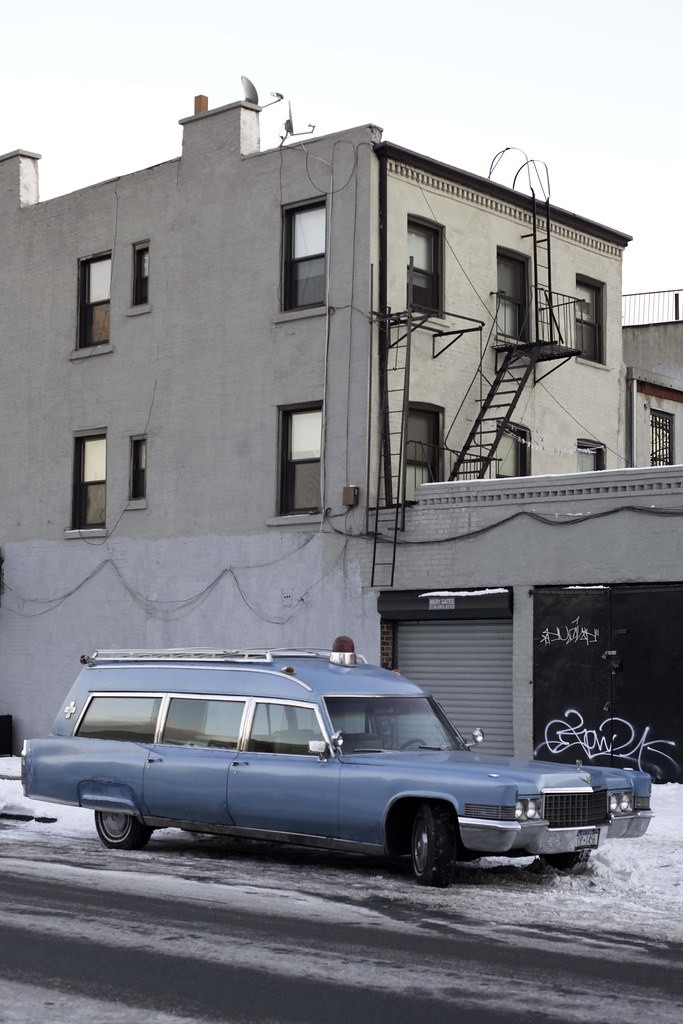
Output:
[272,731,314,753]
[341,731,382,755]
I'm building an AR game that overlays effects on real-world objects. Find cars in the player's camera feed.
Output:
[19,636,655,887]
